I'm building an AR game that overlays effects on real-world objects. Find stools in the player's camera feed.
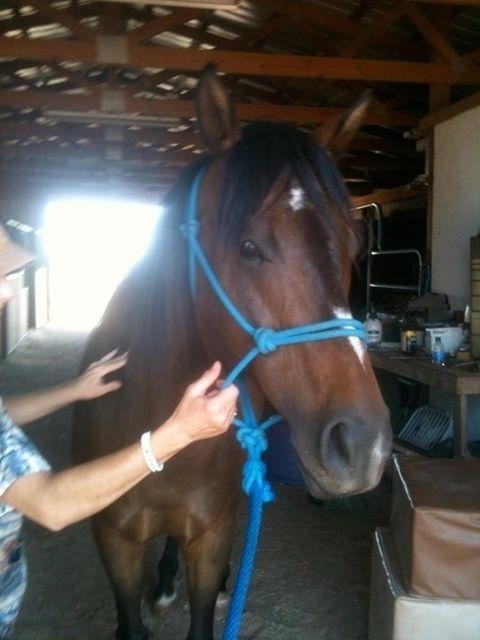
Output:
[367,526,480,640]
[388,451,479,602]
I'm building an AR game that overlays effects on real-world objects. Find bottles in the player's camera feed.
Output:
[433,337,445,367]
[365,310,383,352]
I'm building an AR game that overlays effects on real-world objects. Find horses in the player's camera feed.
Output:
[71,58,395,640]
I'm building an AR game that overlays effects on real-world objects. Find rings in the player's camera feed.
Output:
[232,412,237,416]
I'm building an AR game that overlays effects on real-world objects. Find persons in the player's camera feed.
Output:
[0,237,239,639]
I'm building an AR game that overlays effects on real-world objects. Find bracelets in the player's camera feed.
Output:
[136,429,167,477]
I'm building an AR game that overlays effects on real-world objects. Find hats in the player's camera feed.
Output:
[0,225,38,279]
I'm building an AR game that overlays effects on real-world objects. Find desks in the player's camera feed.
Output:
[366,346,479,458]
